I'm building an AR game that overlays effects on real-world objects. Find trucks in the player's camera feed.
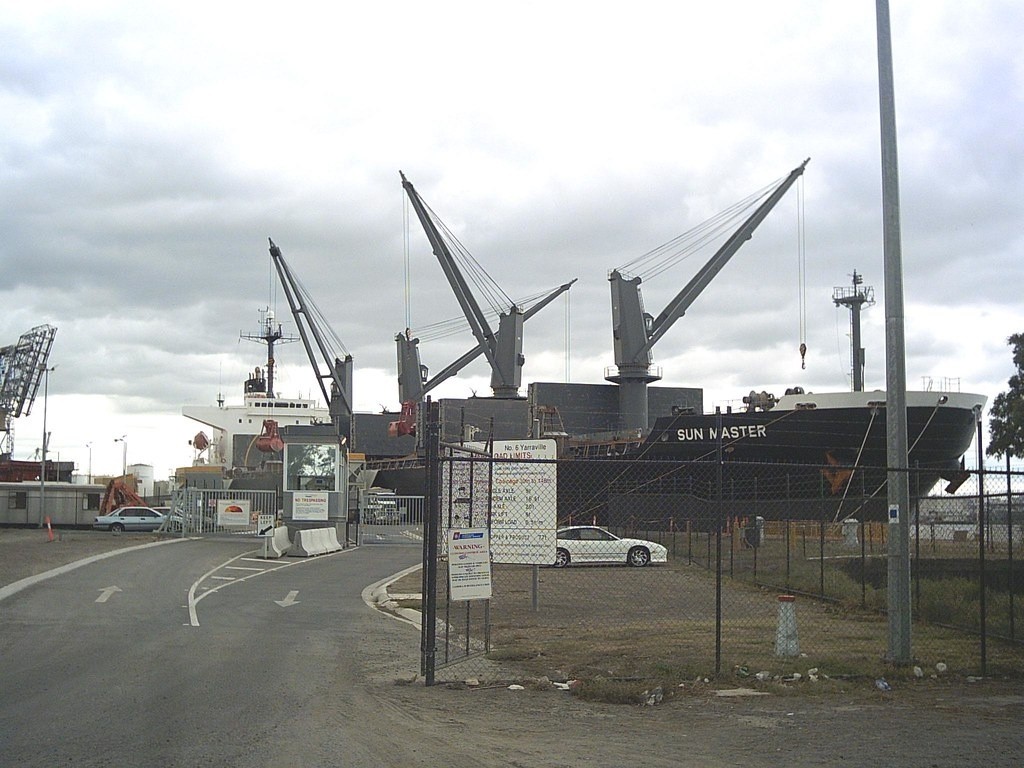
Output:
[349,486,401,525]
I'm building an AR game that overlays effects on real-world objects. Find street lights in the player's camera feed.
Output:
[85,441,93,485]
[114,435,128,488]
[38,366,56,530]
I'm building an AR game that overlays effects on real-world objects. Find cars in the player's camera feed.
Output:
[92,506,212,532]
[553,526,670,570]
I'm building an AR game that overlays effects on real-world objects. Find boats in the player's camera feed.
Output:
[183,156,988,531]
